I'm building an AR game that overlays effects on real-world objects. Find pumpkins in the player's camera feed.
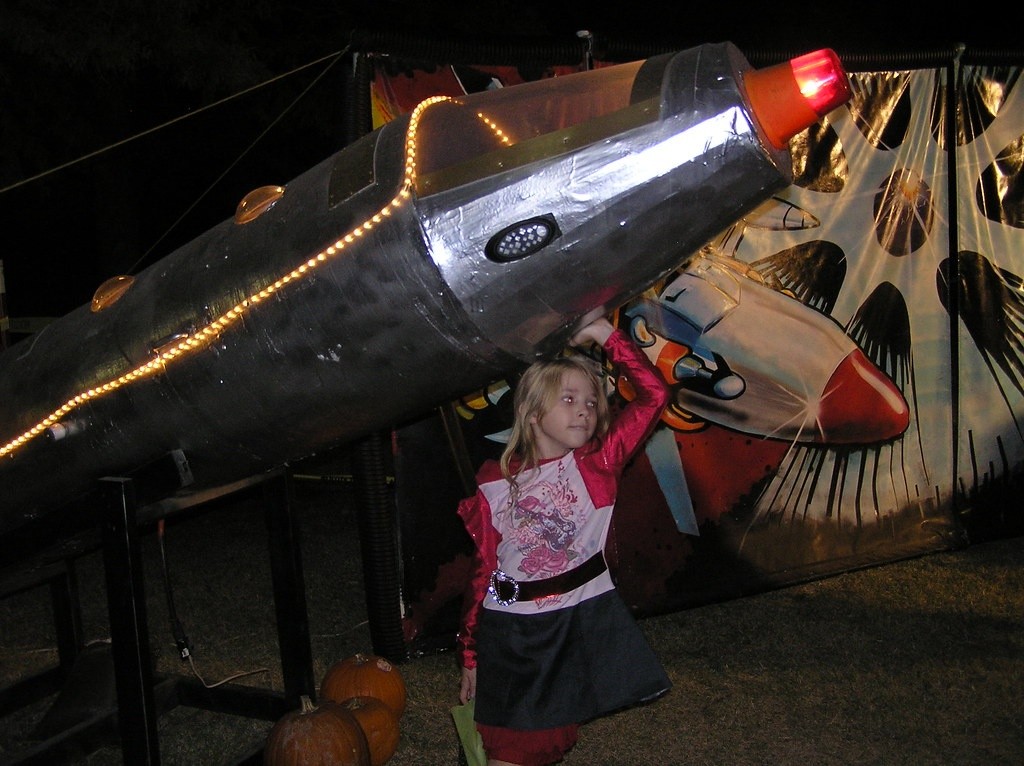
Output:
[265,653,406,766]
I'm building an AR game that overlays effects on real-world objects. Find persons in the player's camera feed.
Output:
[448,317,672,766]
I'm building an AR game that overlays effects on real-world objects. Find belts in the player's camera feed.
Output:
[488,550,607,607]
